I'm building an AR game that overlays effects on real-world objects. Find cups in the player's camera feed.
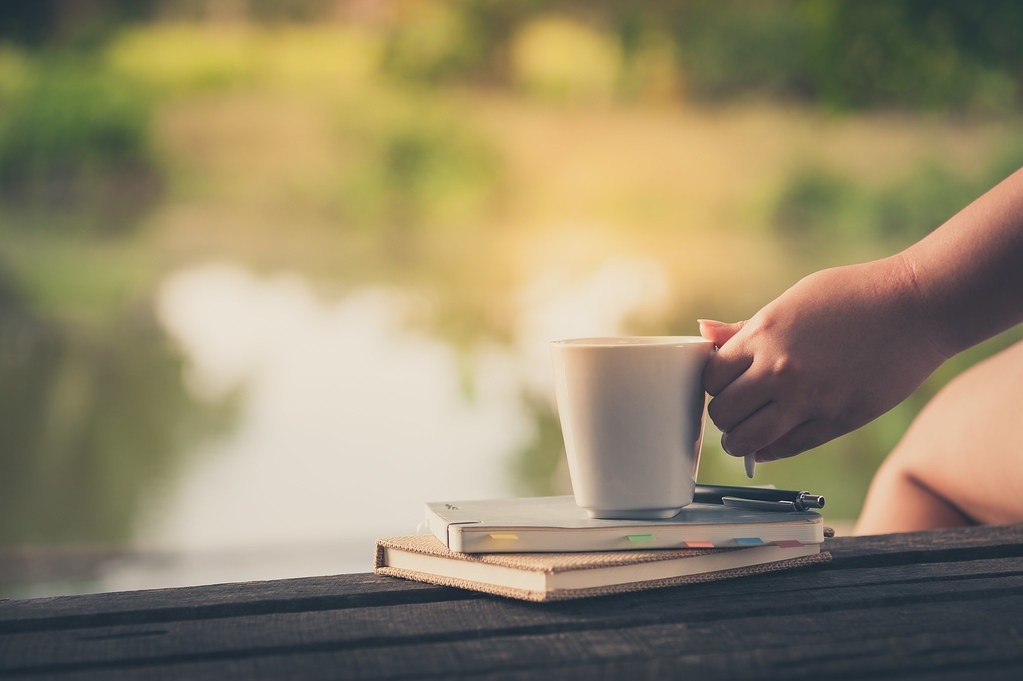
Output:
[546,335,757,520]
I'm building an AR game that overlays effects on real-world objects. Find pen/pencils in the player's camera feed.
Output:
[692,482,827,514]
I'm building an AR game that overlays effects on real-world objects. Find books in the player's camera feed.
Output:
[372,484,832,603]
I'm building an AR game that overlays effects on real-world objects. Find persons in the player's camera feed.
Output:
[695,163,1023,537]
